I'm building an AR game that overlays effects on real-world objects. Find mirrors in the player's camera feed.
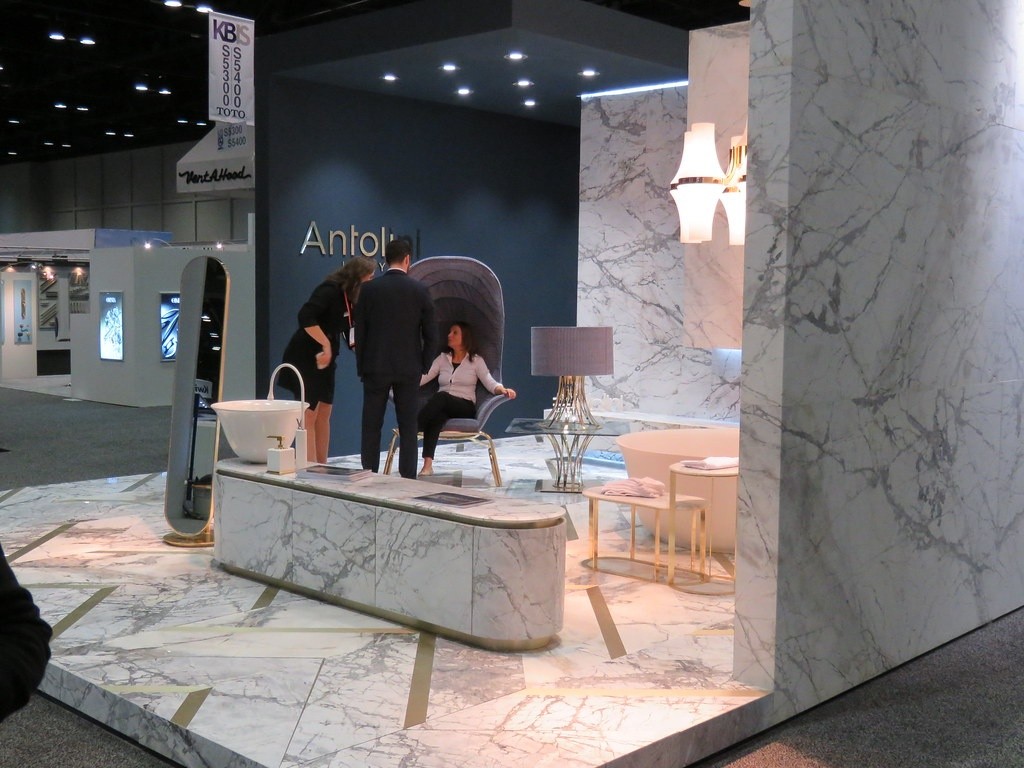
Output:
[162,256,232,548]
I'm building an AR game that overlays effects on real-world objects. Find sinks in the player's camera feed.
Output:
[210,399,310,463]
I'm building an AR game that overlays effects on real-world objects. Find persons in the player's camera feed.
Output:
[351,240,441,480]
[0,541,53,724]
[392,321,517,476]
[277,257,378,464]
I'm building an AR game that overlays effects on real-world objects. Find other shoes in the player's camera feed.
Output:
[392,428,400,436]
[418,467,434,476]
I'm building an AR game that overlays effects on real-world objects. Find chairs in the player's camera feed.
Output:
[384,256,516,487]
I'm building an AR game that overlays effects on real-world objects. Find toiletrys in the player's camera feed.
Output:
[266,435,297,476]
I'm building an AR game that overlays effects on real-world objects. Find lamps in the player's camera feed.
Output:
[47,11,97,50]
[668,119,748,246]
[103,118,137,140]
[217,240,235,249]
[132,64,176,97]
[530,326,613,430]
[43,126,75,148]
[144,237,173,250]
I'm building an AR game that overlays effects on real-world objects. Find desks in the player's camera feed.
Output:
[581,486,714,588]
[505,418,622,494]
[668,457,738,597]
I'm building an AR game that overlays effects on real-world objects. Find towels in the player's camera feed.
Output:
[683,456,739,471]
[602,477,665,499]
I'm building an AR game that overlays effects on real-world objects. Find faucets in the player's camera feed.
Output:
[266,363,309,469]
